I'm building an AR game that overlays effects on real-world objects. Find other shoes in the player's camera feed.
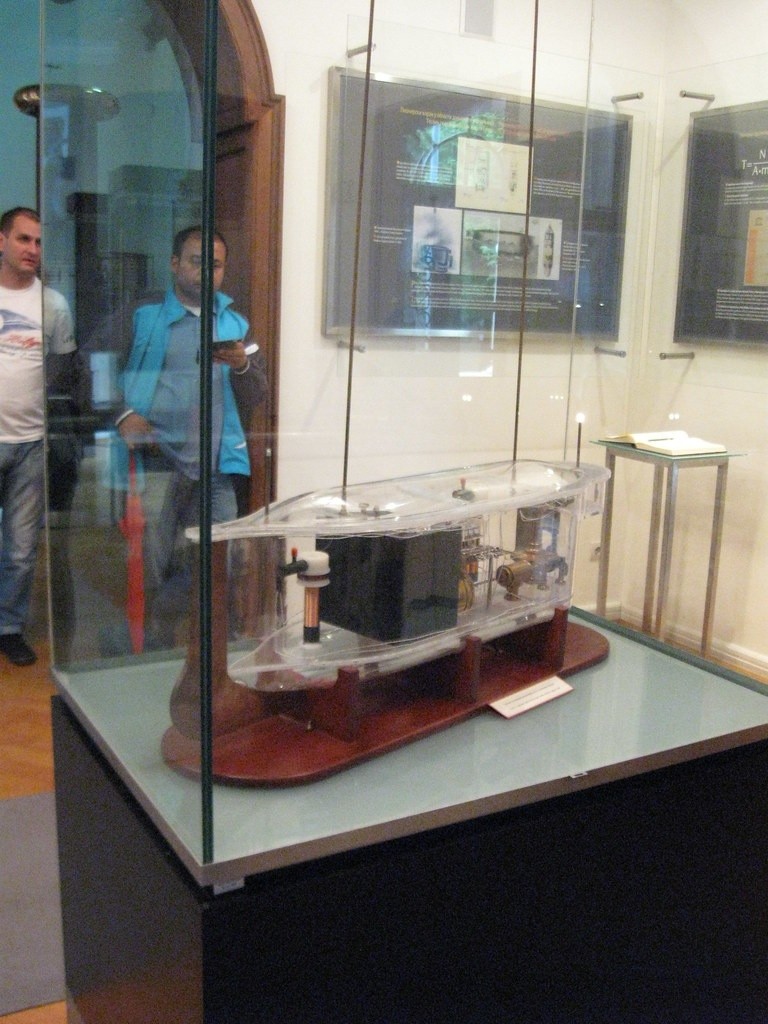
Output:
[1,634,40,665]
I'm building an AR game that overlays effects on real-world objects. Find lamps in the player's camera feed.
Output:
[14,83,121,123]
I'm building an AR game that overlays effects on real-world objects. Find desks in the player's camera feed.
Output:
[46,696,768,1024]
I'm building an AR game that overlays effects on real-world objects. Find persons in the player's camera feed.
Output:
[0,206,78,665]
[71,222,270,662]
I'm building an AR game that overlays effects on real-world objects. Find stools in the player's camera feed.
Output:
[595,432,729,658]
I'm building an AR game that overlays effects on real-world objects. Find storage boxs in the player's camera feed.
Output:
[34,0,768,888]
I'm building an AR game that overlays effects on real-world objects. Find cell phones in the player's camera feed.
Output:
[207,339,241,357]
